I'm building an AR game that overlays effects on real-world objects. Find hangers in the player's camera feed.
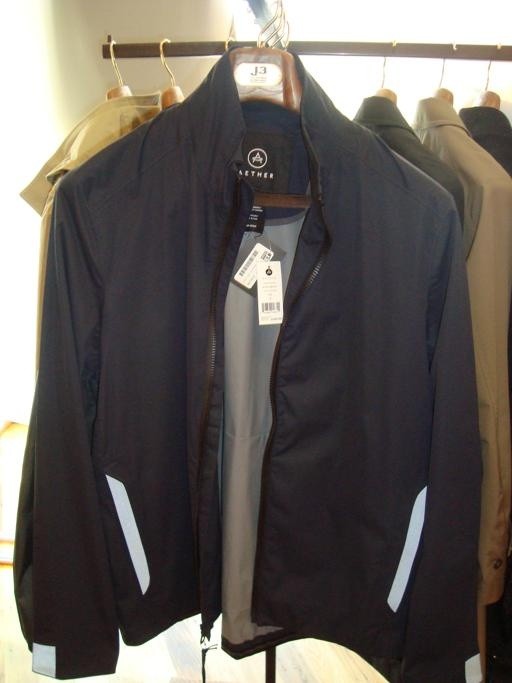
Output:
[228,0,318,210]
